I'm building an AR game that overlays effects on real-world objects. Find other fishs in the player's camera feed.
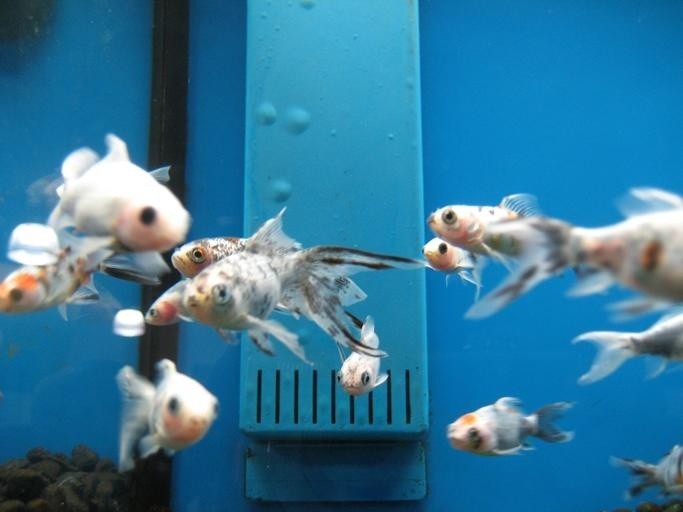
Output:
[0,247,116,316]
[144,210,417,364]
